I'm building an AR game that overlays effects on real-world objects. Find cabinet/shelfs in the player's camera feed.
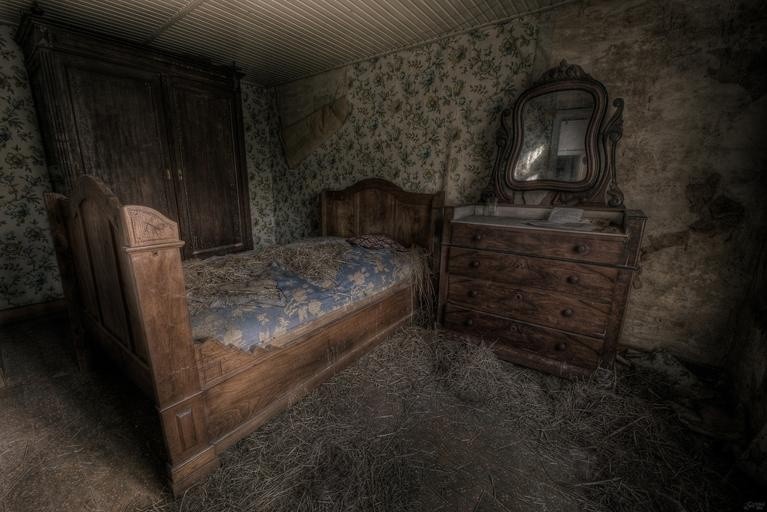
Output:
[432,203,647,390]
[14,13,254,261]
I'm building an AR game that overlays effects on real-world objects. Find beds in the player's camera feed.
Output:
[44,172,446,498]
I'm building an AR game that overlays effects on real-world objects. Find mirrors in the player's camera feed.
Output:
[489,62,623,210]
